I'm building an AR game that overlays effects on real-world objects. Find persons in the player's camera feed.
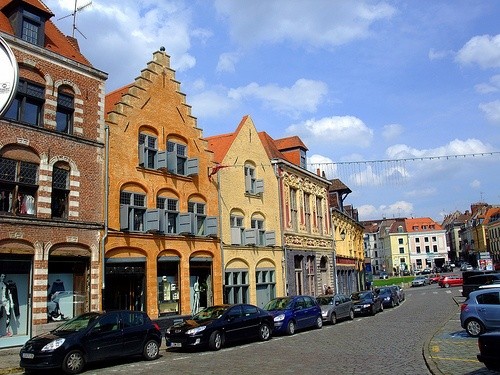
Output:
[326,286,333,295]
[0,273,20,337]
[192,276,201,314]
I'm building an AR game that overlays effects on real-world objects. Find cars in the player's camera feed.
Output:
[165,302,274,352]
[348,284,406,316]
[413,260,474,275]
[261,295,323,337]
[438,274,464,288]
[315,293,355,325]
[412,275,433,287]
[18,308,162,375]
[402,269,410,276]
[429,274,441,283]
[459,278,500,372]
[379,272,388,280]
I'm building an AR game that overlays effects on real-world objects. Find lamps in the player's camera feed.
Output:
[336,230,345,241]
